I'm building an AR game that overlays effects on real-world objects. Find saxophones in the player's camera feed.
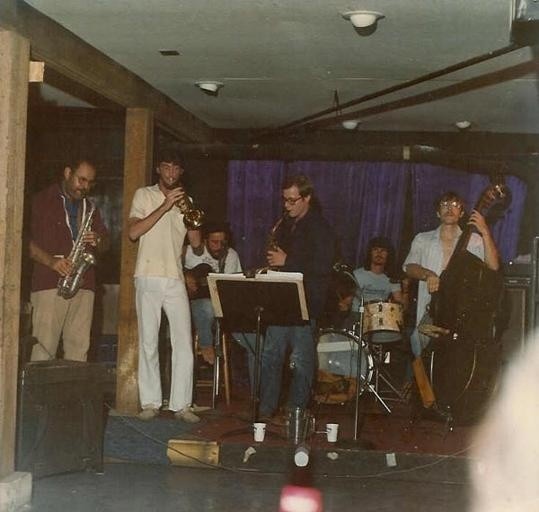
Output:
[258,212,290,272]
[58,190,99,299]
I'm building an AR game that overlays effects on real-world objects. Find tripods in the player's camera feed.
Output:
[221,330,282,441]
[353,354,408,414]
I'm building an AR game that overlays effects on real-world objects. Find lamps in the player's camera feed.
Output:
[341,10,385,31]
[193,79,225,94]
[341,119,362,130]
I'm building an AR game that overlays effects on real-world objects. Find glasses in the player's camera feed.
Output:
[282,196,304,205]
[439,201,461,209]
[162,163,185,176]
[74,171,96,187]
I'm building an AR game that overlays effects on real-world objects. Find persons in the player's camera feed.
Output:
[341,235,413,397]
[252,170,336,420]
[158,263,214,413]
[23,155,109,361]
[403,191,500,356]
[129,149,201,425]
[184,224,243,365]
[469,327,538,511]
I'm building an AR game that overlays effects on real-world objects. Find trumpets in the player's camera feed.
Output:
[170,180,205,229]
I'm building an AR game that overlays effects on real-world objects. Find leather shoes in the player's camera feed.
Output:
[200,346,216,366]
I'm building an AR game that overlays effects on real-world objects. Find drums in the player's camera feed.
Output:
[363,301,402,341]
[315,329,373,405]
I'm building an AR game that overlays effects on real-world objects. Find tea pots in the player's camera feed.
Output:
[288,407,316,445]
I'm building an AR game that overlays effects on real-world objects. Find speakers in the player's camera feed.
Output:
[105,414,186,462]
[18,358,105,477]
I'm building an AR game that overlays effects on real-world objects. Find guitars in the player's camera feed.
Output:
[184,264,258,299]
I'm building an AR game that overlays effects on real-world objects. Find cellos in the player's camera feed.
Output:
[412,183,512,423]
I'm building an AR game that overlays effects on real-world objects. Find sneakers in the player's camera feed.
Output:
[137,408,160,420]
[174,410,201,424]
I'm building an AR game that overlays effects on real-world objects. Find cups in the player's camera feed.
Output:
[253,422,267,442]
[326,423,340,442]
[294,446,309,467]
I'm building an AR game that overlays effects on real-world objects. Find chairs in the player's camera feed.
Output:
[161,265,366,442]
